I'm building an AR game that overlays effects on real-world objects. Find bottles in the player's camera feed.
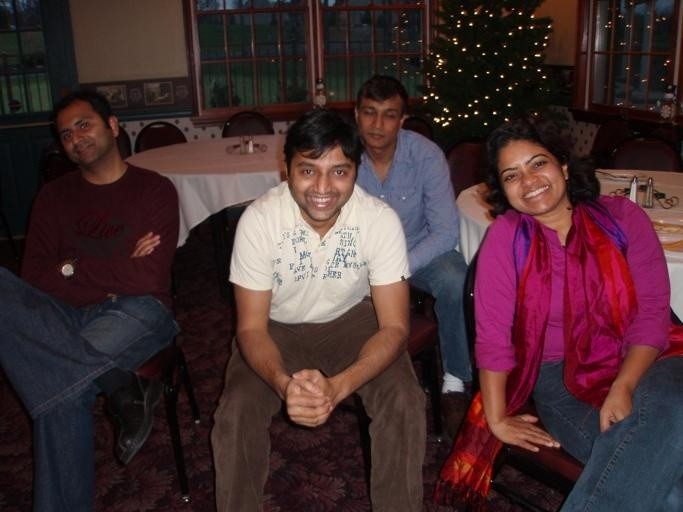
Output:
[643,177,653,208]
[239,134,257,155]
[628,175,637,204]
[313,78,328,109]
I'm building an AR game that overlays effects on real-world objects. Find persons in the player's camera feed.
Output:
[353,75,479,442]
[0,91,180,512]
[473,119,683,512]
[211,110,426,512]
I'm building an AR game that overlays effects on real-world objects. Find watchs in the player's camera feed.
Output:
[61,263,74,280]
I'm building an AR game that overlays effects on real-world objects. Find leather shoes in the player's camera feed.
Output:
[104,371,163,465]
[441,390,470,446]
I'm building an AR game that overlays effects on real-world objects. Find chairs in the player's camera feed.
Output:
[42,110,682,511]
